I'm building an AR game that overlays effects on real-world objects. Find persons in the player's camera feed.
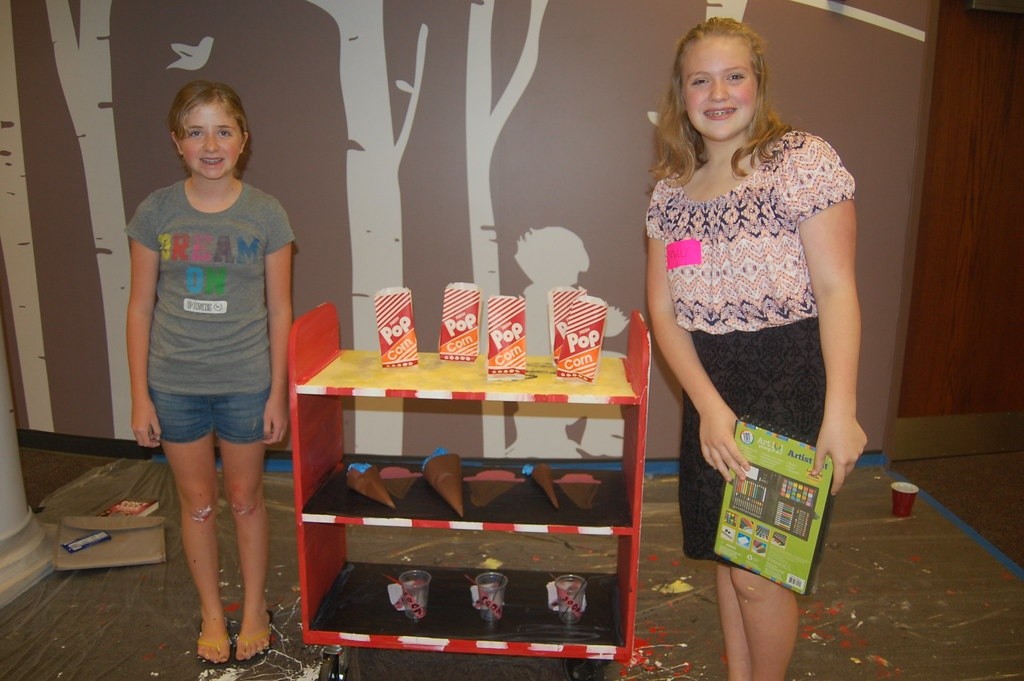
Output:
[644,18,867,681]
[127,79,296,668]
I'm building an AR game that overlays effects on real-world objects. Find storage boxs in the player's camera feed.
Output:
[714,414,834,595]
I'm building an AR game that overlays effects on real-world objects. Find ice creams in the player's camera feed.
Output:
[462,469,526,506]
[345,462,396,509]
[552,473,602,509]
[421,448,463,519]
[521,462,560,508]
[378,465,422,499]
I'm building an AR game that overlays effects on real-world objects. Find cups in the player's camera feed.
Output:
[554,575,587,624]
[399,570,432,619]
[891,481,919,518]
[475,573,508,622]
